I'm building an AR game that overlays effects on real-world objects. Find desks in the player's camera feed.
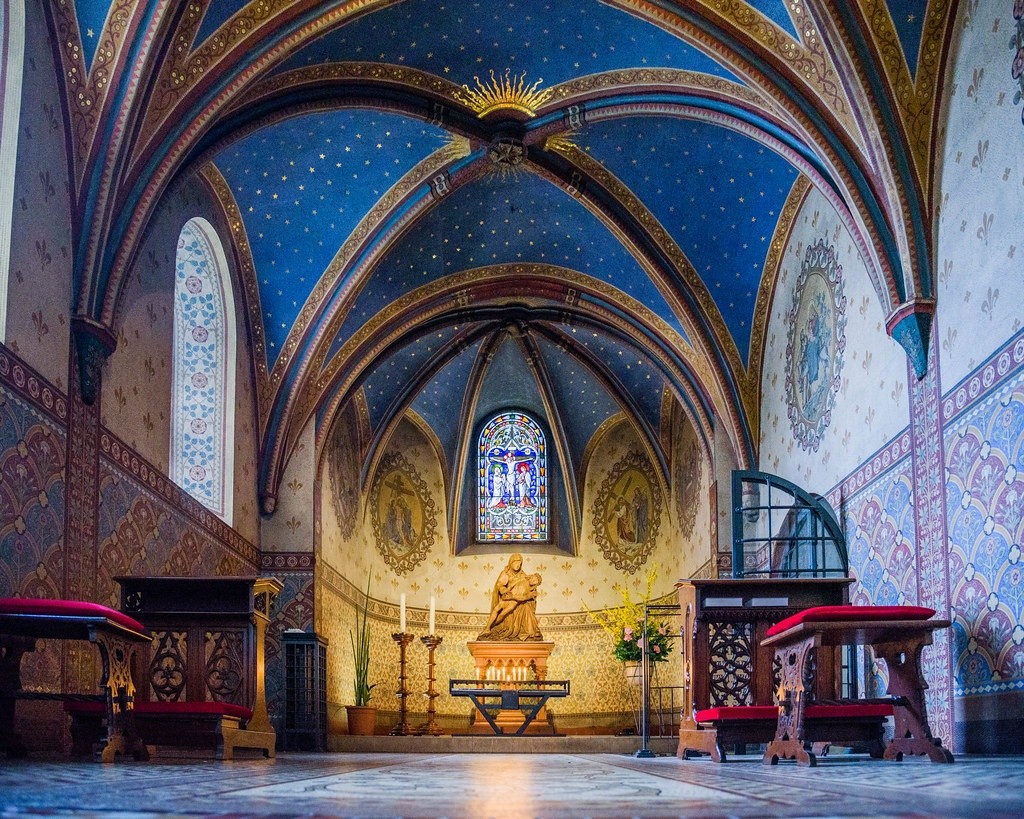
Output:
[282,632,330,752]
[449,679,570,737]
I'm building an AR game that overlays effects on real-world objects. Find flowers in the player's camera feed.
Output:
[578,559,679,663]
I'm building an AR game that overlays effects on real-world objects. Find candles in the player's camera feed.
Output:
[400,593,406,632]
[476,666,527,681]
[429,596,435,636]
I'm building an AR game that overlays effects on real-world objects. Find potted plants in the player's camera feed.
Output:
[330,563,378,736]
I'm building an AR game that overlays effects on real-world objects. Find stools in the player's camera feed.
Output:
[759,606,955,767]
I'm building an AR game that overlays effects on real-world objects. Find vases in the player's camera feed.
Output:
[624,660,654,685]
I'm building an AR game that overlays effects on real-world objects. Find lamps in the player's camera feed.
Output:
[0,598,153,765]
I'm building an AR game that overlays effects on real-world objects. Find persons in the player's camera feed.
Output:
[495,554,540,638]
[478,573,542,637]
